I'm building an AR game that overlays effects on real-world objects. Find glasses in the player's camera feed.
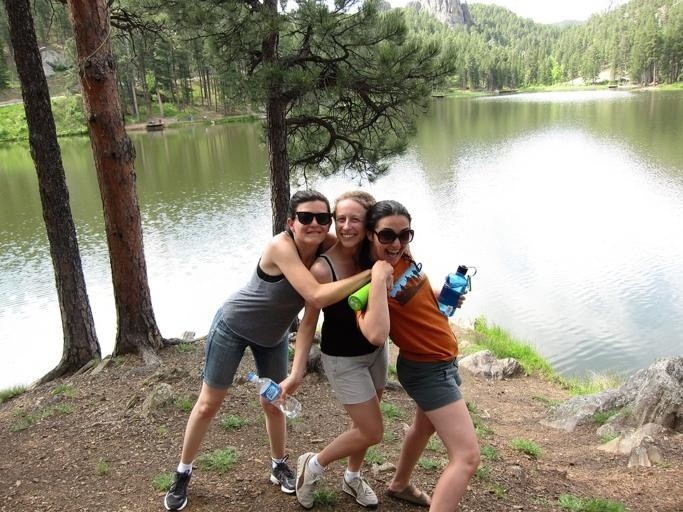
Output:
[373,228,415,244]
[294,211,334,226]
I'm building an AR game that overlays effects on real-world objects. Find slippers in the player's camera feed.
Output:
[384,482,431,507]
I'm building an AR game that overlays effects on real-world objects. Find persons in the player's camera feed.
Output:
[274,191,386,511]
[161,190,396,511]
[353,200,480,511]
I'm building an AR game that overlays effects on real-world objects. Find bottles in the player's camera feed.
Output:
[436,263,478,317]
[346,283,374,311]
[245,372,302,419]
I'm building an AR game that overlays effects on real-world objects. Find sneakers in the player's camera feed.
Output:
[163,469,194,512]
[295,452,320,510]
[341,468,379,508]
[268,460,297,494]
